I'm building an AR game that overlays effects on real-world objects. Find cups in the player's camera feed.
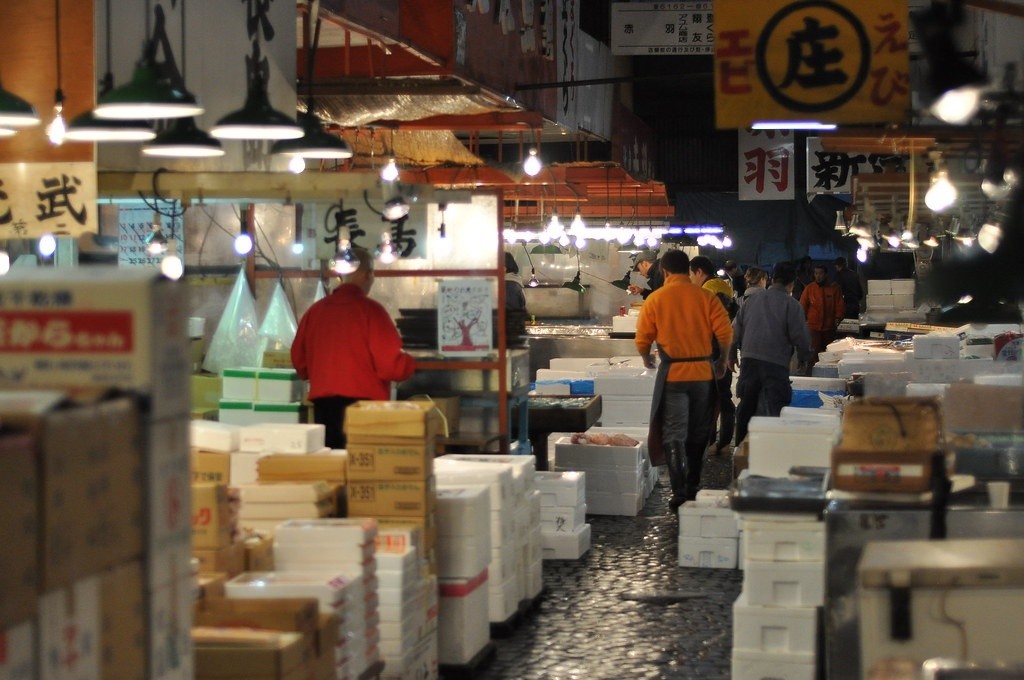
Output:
[987,482,1009,511]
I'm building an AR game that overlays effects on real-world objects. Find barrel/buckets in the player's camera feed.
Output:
[789,376,846,407]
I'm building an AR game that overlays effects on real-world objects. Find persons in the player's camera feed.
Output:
[939,277,1021,326]
[627,248,863,508]
[503,251,532,336]
[291,247,416,450]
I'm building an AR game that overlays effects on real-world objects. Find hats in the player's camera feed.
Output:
[633,250,656,272]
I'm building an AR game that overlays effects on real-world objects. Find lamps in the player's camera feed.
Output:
[0,0,735,293]
[835,0,1024,263]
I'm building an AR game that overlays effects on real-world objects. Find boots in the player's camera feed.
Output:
[687,448,707,499]
[664,441,686,510]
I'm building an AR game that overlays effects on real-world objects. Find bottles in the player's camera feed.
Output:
[530,312,536,326]
[612,302,643,333]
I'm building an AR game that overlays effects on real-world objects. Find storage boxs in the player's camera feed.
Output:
[1,256,1024,680]
